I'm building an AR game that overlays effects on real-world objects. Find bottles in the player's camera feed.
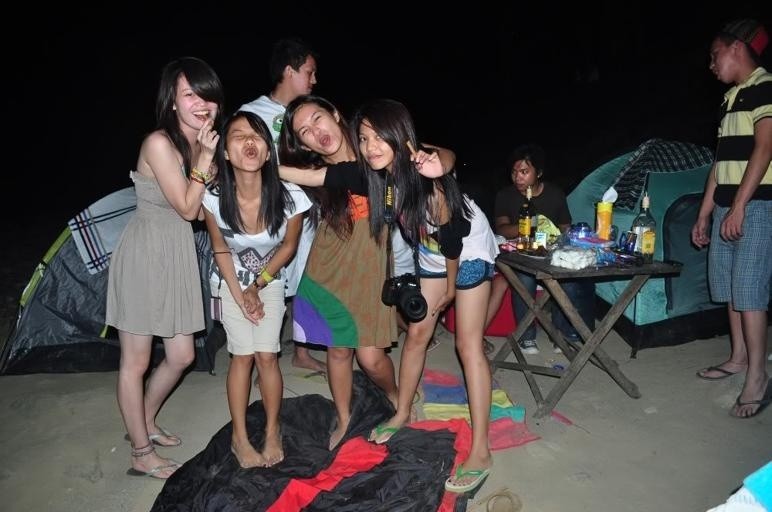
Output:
[518,185,539,245]
[628,191,656,265]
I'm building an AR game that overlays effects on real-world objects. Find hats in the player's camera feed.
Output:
[697,19,769,56]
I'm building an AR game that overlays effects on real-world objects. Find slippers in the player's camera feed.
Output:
[466,488,523,512]
[696,365,739,380]
[444,453,493,492]
[728,377,772,418]
[426,338,442,353]
[366,414,410,445]
[127,457,183,480]
[483,338,494,355]
[124,425,181,447]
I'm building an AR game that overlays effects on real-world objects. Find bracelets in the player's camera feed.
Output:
[191,167,211,184]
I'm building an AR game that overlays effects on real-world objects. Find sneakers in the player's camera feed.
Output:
[547,335,563,354]
[516,338,540,354]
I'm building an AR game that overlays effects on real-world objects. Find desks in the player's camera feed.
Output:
[488,248,683,419]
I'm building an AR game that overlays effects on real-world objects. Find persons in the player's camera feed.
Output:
[693,17,772,419]
[202,94,454,469]
[234,41,328,387]
[351,99,501,491]
[492,143,577,354]
[103,57,228,478]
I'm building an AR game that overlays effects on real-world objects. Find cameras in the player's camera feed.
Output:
[381,273,428,323]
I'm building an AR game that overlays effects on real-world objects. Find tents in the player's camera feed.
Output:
[563,136,772,360]
[0,183,228,376]
